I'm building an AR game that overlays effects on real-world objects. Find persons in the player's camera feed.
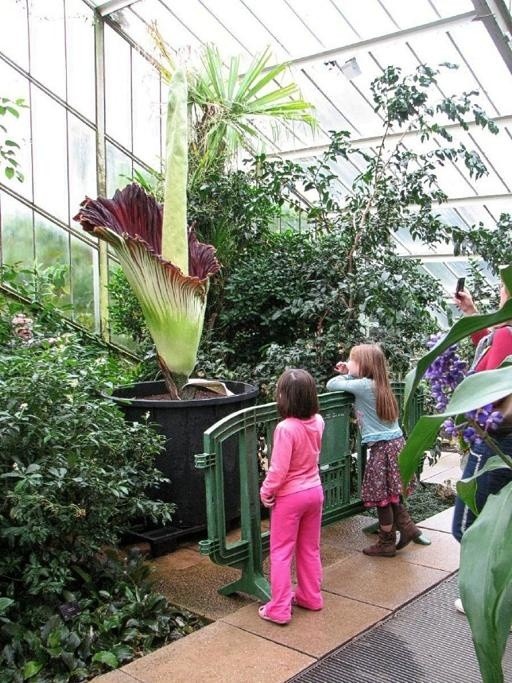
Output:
[455,279,512,616]
[327,343,422,557]
[258,370,325,625]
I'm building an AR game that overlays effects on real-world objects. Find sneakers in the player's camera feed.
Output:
[290,591,323,611]
[258,604,289,625]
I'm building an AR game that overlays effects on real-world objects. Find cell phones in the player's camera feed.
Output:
[456,278,465,298]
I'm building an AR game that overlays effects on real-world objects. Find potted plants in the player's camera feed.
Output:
[73,58,261,529]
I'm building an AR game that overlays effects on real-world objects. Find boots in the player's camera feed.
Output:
[394,515,421,550]
[362,523,397,558]
